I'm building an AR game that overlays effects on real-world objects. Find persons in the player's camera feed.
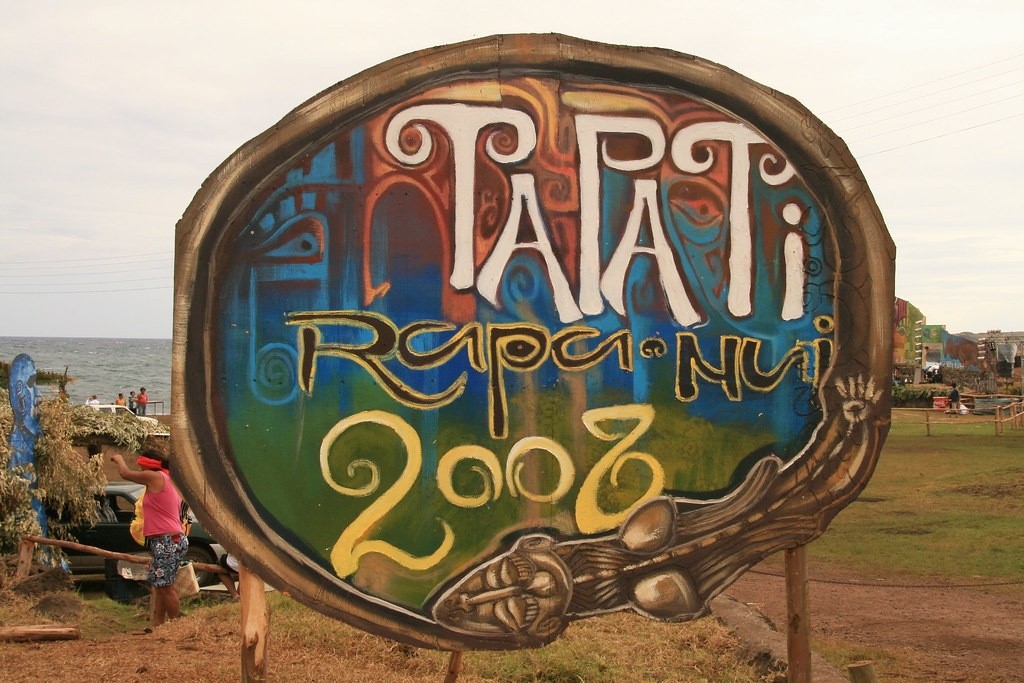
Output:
[129,391,138,414]
[217,553,240,605]
[86,395,99,405]
[110,449,189,634]
[949,382,959,409]
[115,394,126,406]
[136,387,148,416]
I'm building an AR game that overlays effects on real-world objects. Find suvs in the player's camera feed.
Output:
[42,484,241,587]
[87,404,159,427]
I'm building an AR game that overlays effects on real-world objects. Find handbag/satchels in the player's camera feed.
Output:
[173,561,199,598]
